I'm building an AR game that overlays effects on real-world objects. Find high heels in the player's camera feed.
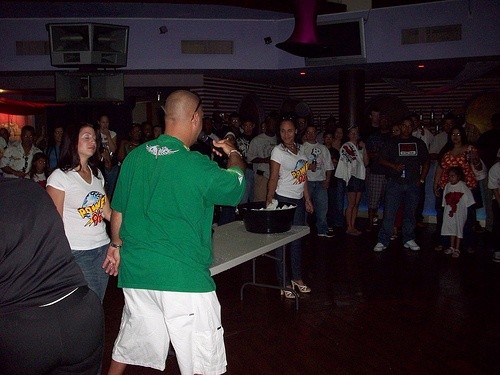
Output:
[281,286,299,298]
[290,280,311,292]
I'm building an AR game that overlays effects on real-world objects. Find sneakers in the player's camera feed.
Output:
[403,239,420,250]
[374,242,387,251]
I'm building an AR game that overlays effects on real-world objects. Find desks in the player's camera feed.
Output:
[209,220,311,316]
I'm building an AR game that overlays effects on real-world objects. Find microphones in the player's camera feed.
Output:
[198,131,229,160]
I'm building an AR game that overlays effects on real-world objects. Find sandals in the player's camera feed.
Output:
[451,248,460,258]
[444,247,455,254]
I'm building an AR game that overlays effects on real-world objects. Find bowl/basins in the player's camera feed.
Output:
[238,202,298,233]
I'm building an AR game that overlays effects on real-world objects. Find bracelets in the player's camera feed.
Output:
[305,199,311,202]
[109,242,121,248]
[228,149,242,158]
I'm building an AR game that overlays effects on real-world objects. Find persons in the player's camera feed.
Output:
[0,177,105,375]
[266,116,314,298]
[46,123,113,304]
[0,110,500,262]
[102,89,247,375]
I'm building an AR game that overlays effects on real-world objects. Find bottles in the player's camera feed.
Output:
[402,165,406,178]
[312,158,316,171]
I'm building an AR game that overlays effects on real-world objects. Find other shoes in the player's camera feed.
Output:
[318,232,335,238]
[346,230,360,236]
[492,251,500,262]
[355,230,362,234]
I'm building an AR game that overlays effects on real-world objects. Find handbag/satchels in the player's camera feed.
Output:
[466,145,488,181]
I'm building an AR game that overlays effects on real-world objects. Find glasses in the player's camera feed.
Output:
[182,89,202,123]
